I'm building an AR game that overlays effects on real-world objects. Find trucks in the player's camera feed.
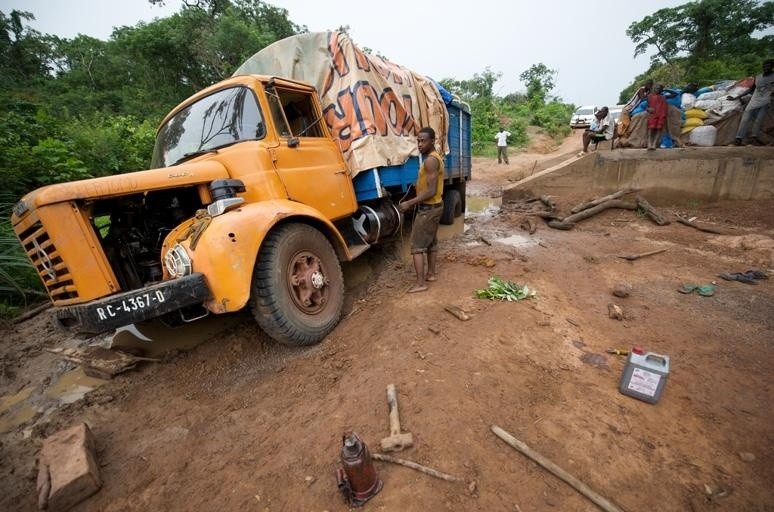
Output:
[3,24,482,364]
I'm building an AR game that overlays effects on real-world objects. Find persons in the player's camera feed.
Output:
[396,127,444,294]
[726,59,773,146]
[577,106,615,158]
[616,79,679,151]
[495,126,511,164]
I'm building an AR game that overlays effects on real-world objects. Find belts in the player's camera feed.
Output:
[417,203,442,210]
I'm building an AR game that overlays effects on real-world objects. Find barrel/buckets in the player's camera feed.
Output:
[619,346,669,406]
[350,199,402,243]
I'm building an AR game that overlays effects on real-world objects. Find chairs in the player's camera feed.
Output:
[281,101,309,137]
[594,123,618,151]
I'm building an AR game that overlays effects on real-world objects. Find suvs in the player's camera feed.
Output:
[569,103,598,129]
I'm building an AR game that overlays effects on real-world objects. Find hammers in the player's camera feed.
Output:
[380,383,414,452]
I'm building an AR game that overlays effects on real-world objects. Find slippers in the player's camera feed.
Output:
[678,284,716,297]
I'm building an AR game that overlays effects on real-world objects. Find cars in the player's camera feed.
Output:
[608,104,626,125]
